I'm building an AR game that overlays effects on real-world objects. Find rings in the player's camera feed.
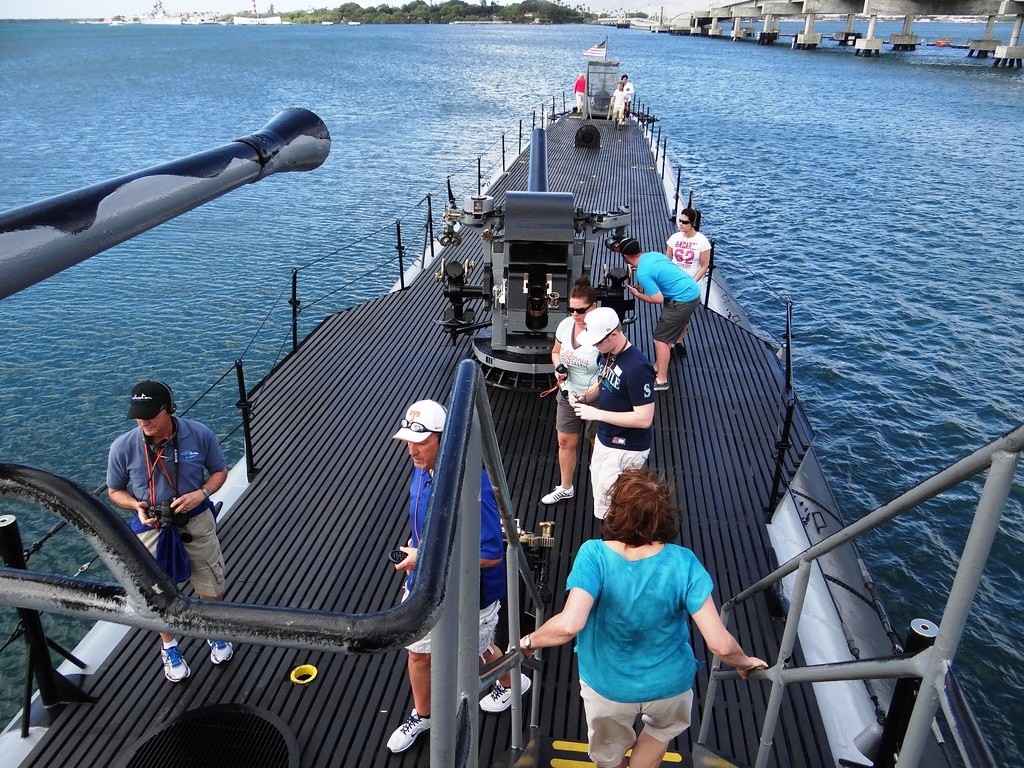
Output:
[184,507,186,511]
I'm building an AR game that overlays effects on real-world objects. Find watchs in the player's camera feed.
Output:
[524,634,533,650]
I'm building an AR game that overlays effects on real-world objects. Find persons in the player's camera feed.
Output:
[574,73,586,114]
[666,209,712,348]
[619,238,701,391]
[610,82,629,129]
[541,278,600,504]
[106,381,233,682]
[621,75,634,119]
[387,399,531,753]
[575,307,656,519]
[520,468,768,768]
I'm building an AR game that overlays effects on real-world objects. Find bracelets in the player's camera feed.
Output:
[201,487,209,497]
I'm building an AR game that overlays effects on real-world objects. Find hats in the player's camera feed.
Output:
[576,307,620,348]
[392,398,449,443]
[126,379,173,419]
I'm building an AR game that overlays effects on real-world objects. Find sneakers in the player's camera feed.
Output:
[479,672,531,712]
[160,642,191,683]
[542,485,574,504]
[654,378,670,390]
[386,708,431,753]
[206,636,233,664]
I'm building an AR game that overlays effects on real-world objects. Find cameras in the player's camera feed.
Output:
[147,500,177,530]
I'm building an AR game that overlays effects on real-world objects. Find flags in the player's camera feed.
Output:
[583,40,605,56]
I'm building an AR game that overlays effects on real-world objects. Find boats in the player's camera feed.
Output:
[70,0,298,26]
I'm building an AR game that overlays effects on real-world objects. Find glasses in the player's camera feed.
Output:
[567,302,593,315]
[679,218,692,224]
[399,418,445,434]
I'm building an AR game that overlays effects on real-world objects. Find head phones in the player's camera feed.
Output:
[158,381,177,414]
[692,210,697,227]
[621,240,637,257]
[142,415,179,449]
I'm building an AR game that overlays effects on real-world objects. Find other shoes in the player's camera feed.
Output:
[675,343,686,354]
[669,347,674,359]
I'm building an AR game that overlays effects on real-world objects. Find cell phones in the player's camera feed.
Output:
[555,363,569,380]
[389,549,408,563]
